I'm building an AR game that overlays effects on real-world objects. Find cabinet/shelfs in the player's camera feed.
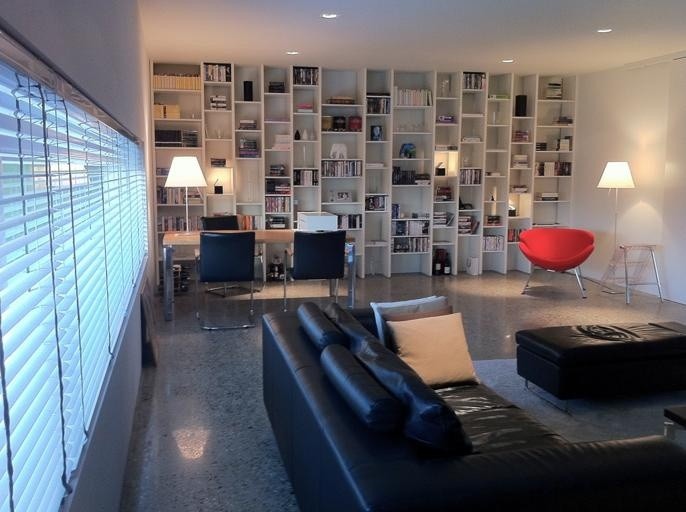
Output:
[152,62,579,286]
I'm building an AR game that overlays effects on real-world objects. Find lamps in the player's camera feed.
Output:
[164,156,207,238]
[597,160,635,295]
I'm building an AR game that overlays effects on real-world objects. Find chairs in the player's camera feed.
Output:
[201,214,266,297]
[281,231,345,313]
[195,232,256,330]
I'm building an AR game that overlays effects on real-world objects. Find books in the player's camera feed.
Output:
[207,93,227,109]
[392,166,432,185]
[237,138,257,156]
[294,67,318,86]
[507,227,526,241]
[294,170,318,186]
[435,142,459,151]
[535,140,547,151]
[267,216,288,229]
[206,64,232,82]
[155,130,198,148]
[458,216,480,236]
[157,183,204,206]
[392,201,402,218]
[512,127,530,143]
[534,158,571,177]
[552,114,576,126]
[153,71,201,91]
[545,75,568,101]
[158,215,206,231]
[154,102,181,119]
[337,213,362,229]
[391,221,430,237]
[433,183,452,201]
[459,169,480,185]
[393,238,428,252]
[265,178,291,196]
[271,132,290,150]
[364,196,388,211]
[237,212,264,230]
[432,209,458,229]
[485,215,503,227]
[321,161,362,177]
[483,236,504,251]
[266,198,291,213]
[463,71,488,90]
[269,164,286,176]
[267,79,286,94]
[512,152,528,168]
[235,116,258,129]
[326,95,356,104]
[366,99,390,114]
[295,102,313,113]
[536,191,559,201]
[394,85,434,107]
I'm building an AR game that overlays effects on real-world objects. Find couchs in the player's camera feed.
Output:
[516,323,685,411]
[521,228,595,299]
[261,305,685,512]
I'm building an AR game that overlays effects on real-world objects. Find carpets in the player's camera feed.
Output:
[471,360,686,448]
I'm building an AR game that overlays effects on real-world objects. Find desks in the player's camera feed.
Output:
[163,231,356,321]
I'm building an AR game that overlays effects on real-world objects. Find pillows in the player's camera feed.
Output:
[377,297,451,312]
[369,295,438,346]
[386,313,481,386]
[382,305,452,321]
